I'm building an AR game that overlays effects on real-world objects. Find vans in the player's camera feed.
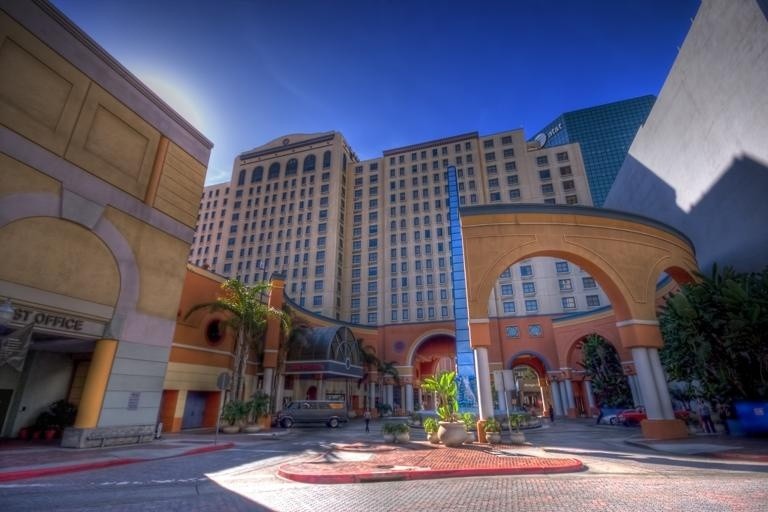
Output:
[277,399,346,429]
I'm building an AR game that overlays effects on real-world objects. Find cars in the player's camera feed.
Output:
[600,406,690,426]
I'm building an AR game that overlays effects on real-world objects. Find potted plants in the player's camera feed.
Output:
[510,413,526,445]
[222,400,247,434]
[383,422,410,444]
[243,390,269,432]
[414,415,421,428]
[463,413,475,443]
[423,417,441,443]
[420,371,467,446]
[483,418,501,444]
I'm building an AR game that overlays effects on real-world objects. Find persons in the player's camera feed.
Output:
[695,400,707,433]
[550,404,554,421]
[700,399,715,433]
[364,407,372,432]
[718,406,726,422]
